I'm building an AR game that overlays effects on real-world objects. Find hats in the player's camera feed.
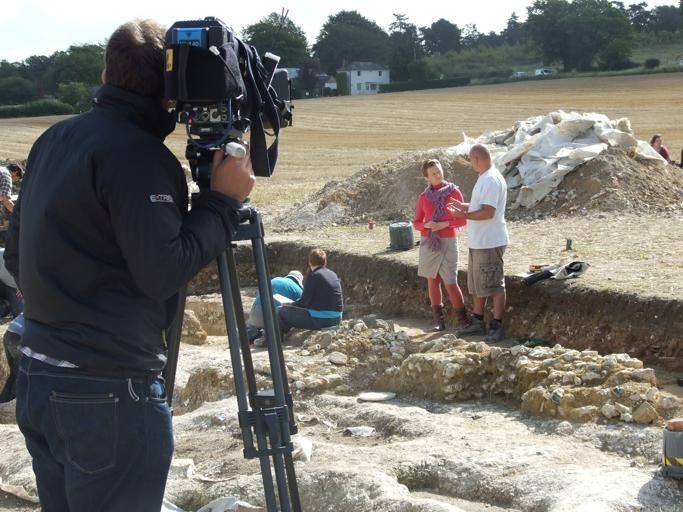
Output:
[511,68,553,80]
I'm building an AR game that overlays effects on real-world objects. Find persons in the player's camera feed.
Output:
[0,246,24,316]
[0,308,26,403]
[412,158,471,332]
[274,247,344,346]
[242,269,305,346]
[445,143,511,343]
[0,17,255,511]
[0,164,24,215]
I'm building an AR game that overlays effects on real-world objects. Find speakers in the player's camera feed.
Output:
[425,303,446,332]
[484,320,504,345]
[456,317,484,337]
[450,309,469,332]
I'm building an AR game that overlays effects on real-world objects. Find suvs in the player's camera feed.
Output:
[388,220,414,250]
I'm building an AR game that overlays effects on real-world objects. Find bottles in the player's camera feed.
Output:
[285,269,306,290]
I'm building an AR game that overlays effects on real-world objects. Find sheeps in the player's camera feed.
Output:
[661,425,683,480]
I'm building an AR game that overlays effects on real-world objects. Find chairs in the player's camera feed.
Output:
[253,336,270,348]
[0,375,17,403]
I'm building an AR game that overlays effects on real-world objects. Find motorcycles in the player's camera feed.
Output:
[181,125,301,512]
